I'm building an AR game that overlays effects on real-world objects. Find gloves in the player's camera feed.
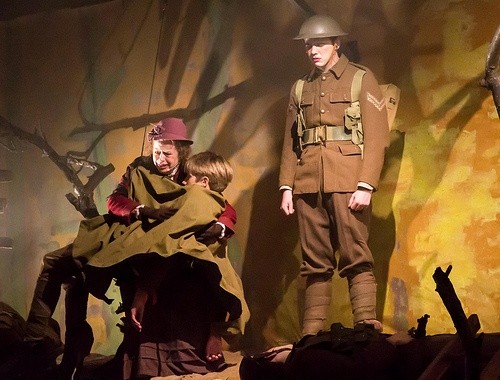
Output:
[138,205,179,223]
[195,223,224,242]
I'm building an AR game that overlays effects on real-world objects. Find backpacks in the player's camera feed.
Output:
[378,82,401,132]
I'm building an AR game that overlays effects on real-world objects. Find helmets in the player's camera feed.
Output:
[291,15,350,40]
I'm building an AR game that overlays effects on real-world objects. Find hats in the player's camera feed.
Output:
[148,117,195,145]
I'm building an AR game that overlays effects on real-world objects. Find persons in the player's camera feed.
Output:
[278,17,390,336]
[106,117,239,380]
[11,153,234,372]
[239,319,500,380]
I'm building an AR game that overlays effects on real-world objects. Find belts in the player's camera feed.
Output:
[302,125,353,145]
